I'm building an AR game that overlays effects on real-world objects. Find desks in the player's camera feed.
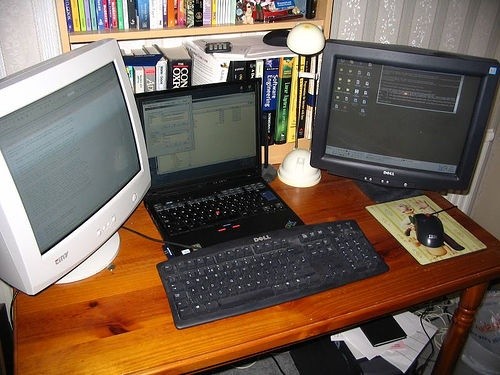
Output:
[13,162,499,375]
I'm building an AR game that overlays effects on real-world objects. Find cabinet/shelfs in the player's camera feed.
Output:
[54,0,335,166]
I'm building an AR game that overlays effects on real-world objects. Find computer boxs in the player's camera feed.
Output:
[289,334,418,375]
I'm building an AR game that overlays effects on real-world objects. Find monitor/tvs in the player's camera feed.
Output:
[0,38,151,296]
[310,38,500,202]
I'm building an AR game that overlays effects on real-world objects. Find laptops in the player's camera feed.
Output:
[134,78,305,257]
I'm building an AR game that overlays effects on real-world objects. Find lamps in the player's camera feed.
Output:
[276,22,327,187]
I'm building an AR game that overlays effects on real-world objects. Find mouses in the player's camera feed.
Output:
[413,213,444,248]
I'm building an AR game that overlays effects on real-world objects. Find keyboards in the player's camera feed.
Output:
[156,220,389,330]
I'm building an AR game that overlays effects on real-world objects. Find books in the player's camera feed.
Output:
[357,315,407,347]
[66,42,324,145]
[61,2,318,33]
